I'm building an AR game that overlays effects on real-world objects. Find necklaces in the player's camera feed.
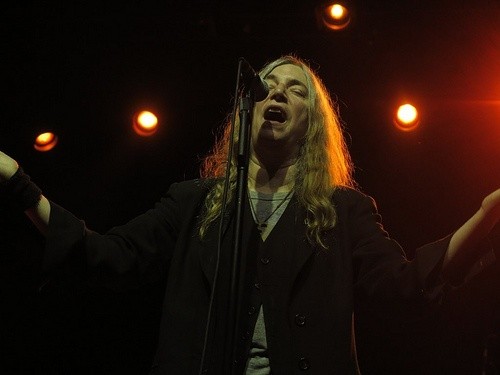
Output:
[246,187,294,233]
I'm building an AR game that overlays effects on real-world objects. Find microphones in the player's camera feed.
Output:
[240,56,270,102]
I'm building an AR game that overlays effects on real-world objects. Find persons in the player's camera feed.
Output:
[1,55,500,375]
